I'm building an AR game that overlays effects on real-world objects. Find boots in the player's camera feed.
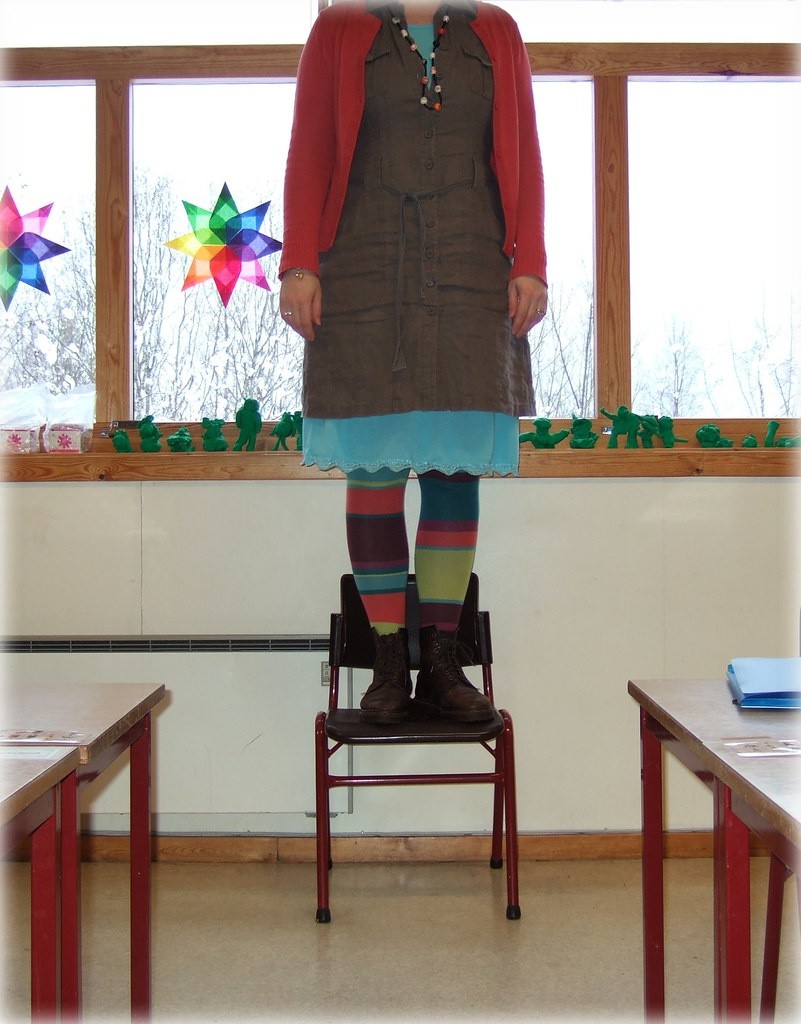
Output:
[358,628,412,724]
[412,624,495,722]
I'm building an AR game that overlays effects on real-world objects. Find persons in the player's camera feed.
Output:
[278,1,549,726]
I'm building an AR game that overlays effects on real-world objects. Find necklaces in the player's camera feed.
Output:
[296,269,303,279]
[387,6,449,110]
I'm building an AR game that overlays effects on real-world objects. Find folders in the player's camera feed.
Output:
[722,655,801,710]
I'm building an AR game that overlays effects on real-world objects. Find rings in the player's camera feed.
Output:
[284,311,291,316]
[536,309,543,313]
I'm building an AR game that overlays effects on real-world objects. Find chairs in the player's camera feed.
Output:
[315,572,521,923]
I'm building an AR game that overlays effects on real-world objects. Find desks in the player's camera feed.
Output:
[0,744,80,1024]
[627,678,801,1024]
[698,742,801,1024]
[0,682,166,1023]
